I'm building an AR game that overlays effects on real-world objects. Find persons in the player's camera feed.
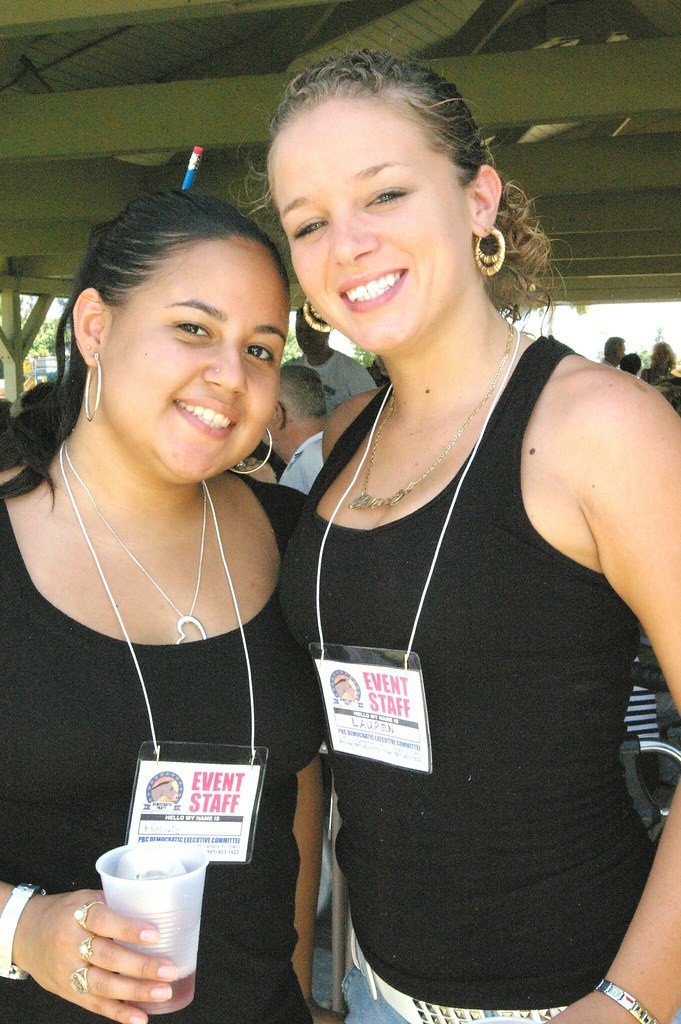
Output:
[229,42,681,1024]
[0,304,681,496]
[0,187,348,1024]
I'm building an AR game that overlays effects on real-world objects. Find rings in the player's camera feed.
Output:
[78,935,96,963]
[73,901,106,931]
[69,964,93,994]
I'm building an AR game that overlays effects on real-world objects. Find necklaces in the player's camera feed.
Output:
[62,441,208,645]
[349,325,514,510]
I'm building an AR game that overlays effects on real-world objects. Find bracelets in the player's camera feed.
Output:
[595,978,659,1024]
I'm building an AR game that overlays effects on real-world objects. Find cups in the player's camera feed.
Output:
[95,841,210,1015]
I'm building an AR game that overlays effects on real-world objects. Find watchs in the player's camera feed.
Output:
[0,882,46,980]
